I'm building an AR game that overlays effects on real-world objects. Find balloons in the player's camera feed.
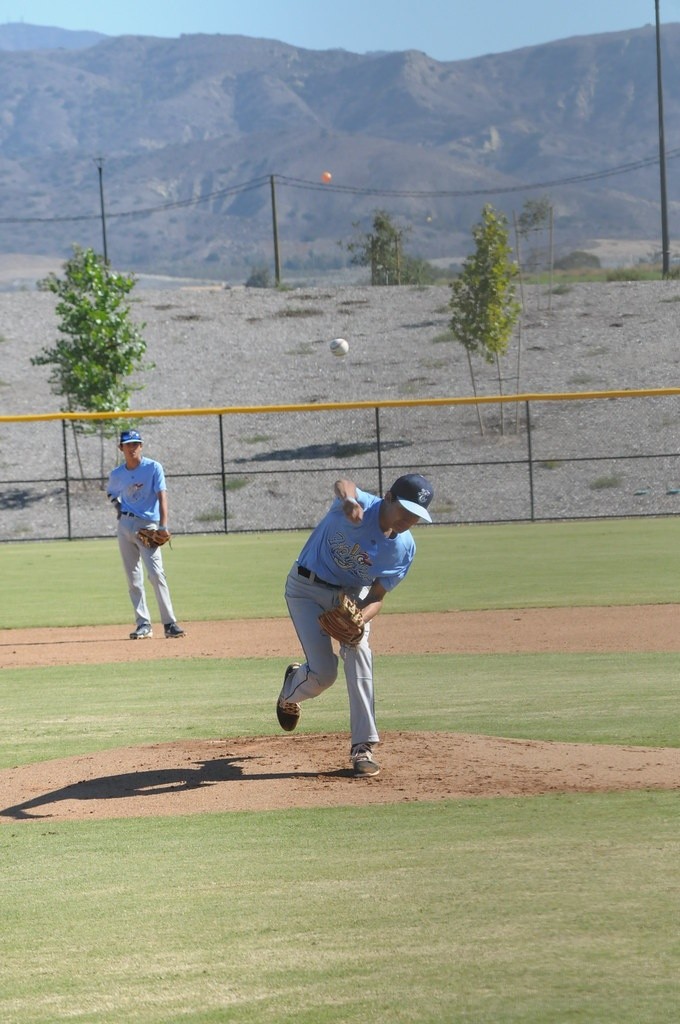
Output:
[321,172,332,182]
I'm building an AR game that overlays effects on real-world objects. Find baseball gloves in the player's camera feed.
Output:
[137,527,171,548]
[316,592,366,647]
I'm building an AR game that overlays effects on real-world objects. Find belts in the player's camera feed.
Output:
[121,512,140,518]
[298,566,342,588]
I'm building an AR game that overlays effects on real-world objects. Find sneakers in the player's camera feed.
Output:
[164,622,184,636]
[276,662,302,731]
[350,741,379,777]
[130,624,153,638]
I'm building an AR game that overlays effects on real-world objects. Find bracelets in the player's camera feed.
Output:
[159,523,168,530]
[342,497,356,504]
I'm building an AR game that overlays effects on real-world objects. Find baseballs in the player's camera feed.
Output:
[330,338,348,356]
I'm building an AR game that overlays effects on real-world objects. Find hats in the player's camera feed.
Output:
[391,473,433,523]
[120,430,142,444]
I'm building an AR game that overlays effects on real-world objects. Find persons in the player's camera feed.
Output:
[107,430,187,640]
[276,472,434,775]
